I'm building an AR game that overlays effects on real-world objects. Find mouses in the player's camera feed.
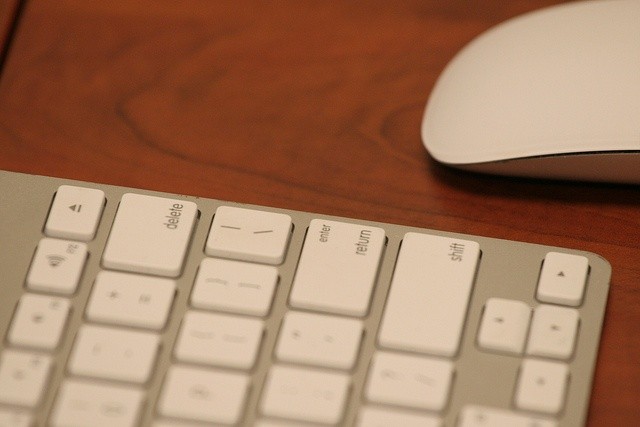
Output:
[420,0,635,183]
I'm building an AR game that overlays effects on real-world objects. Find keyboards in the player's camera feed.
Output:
[1,168,612,427]
